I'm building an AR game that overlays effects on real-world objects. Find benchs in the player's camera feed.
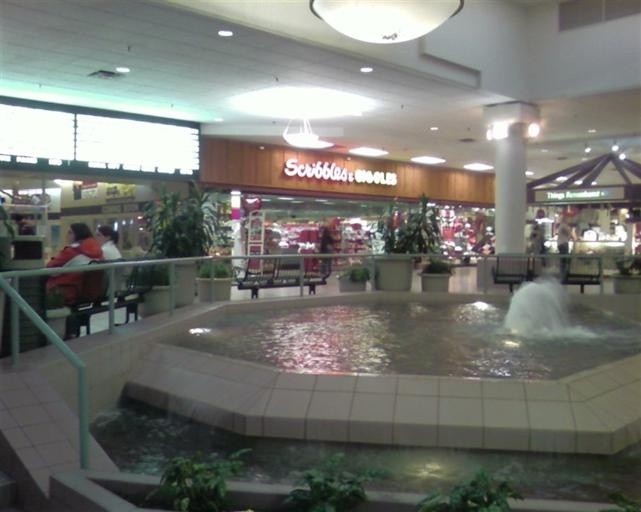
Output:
[235,254,333,300]
[70,257,154,339]
[491,253,604,294]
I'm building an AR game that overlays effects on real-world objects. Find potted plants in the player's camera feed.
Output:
[336,193,455,293]
[131,168,242,318]
[44,288,69,341]
[611,250,640,296]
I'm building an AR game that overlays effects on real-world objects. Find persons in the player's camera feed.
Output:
[94,223,124,297]
[531,209,546,267]
[45,221,104,307]
[10,211,37,235]
[319,225,340,282]
[558,214,579,272]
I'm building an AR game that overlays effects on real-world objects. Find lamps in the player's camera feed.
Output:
[309,0,466,45]
